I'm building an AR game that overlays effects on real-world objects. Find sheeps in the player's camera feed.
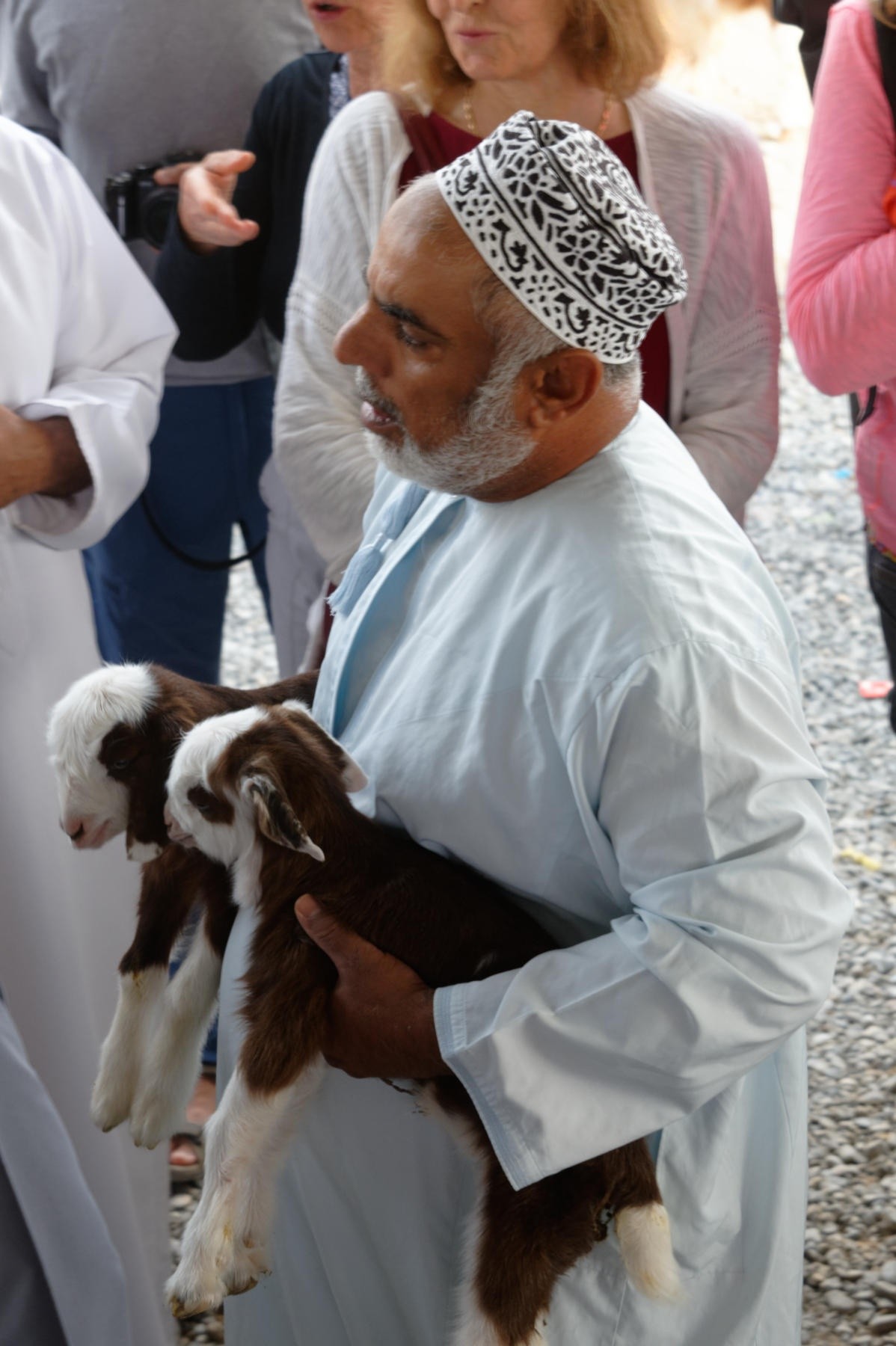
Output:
[156,691,690,1345]
[40,652,326,1154]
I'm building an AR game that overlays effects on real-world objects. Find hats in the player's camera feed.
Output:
[434,109,688,364]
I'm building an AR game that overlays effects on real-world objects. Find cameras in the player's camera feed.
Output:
[105,148,208,247]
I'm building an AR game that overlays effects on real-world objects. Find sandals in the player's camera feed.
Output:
[166,1065,218,1182]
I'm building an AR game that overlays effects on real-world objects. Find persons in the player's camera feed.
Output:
[785,0,896,727]
[152,0,395,362]
[0,0,325,1181]
[210,110,853,1346]
[0,115,178,1346]
[272,0,740,588]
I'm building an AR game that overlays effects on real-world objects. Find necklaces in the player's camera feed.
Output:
[462,82,614,140]
